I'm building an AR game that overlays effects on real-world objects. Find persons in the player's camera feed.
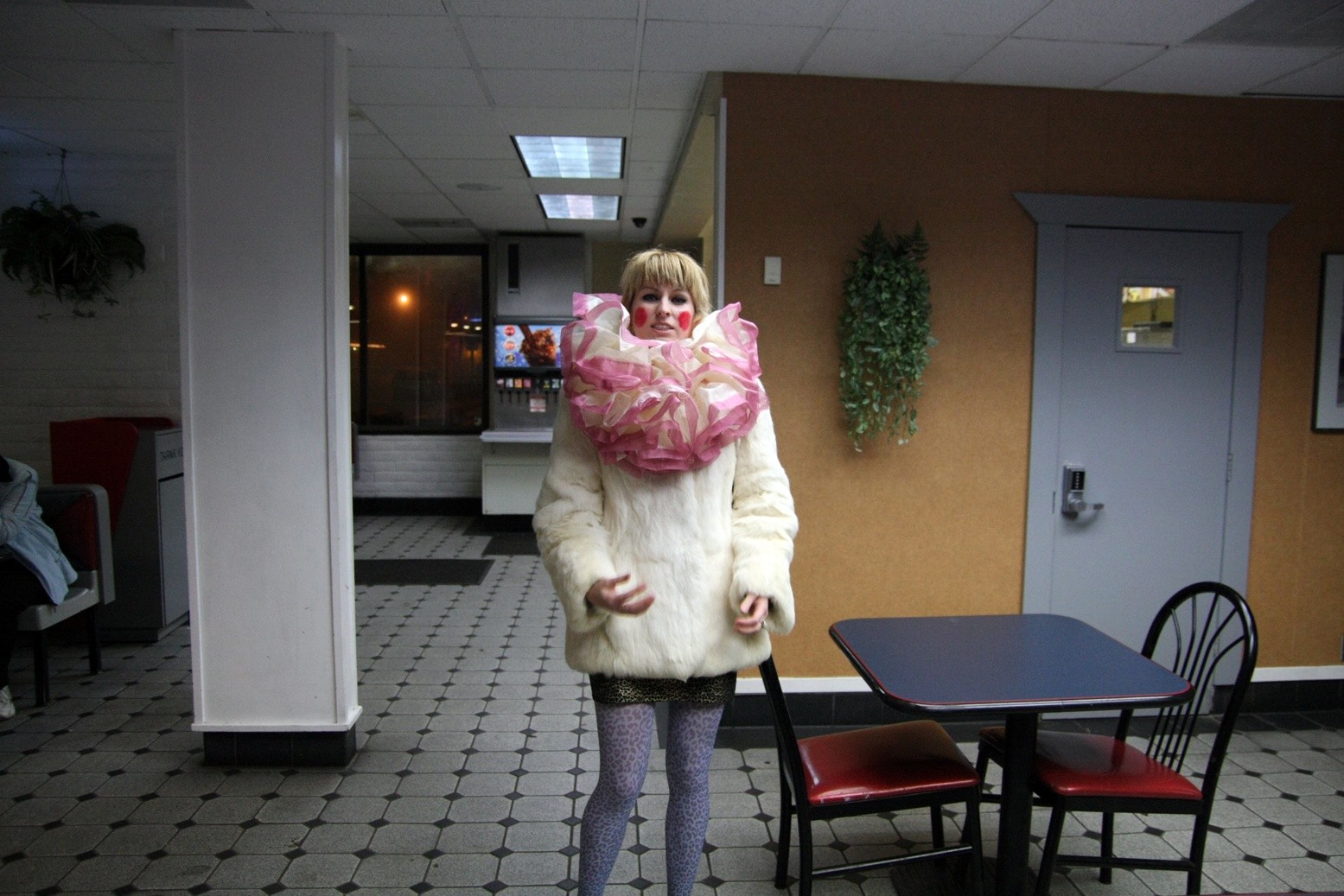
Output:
[0,454,80,728]
[533,251,800,896]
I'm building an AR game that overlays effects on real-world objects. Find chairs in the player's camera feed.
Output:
[758,656,984,896]
[956,581,1258,896]
[17,483,115,706]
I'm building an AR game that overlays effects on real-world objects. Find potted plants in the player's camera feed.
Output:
[0,154,146,320]
[836,221,939,453]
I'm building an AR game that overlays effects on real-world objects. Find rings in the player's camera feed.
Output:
[761,619,767,628]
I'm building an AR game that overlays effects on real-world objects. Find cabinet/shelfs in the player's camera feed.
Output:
[49,423,189,643]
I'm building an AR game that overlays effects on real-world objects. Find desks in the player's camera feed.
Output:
[830,613,1195,896]
